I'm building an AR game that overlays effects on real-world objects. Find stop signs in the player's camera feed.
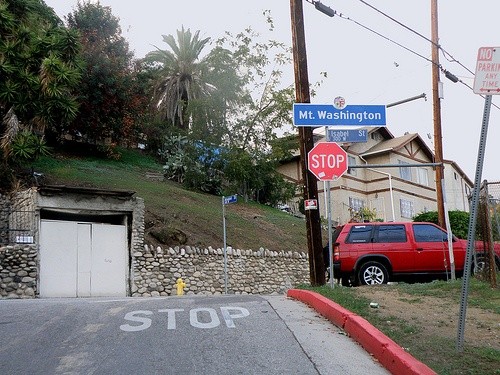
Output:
[308,142,348,182]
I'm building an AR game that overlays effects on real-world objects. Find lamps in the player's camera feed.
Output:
[33,172,44,186]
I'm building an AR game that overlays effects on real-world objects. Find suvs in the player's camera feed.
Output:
[322,220,500,286]
